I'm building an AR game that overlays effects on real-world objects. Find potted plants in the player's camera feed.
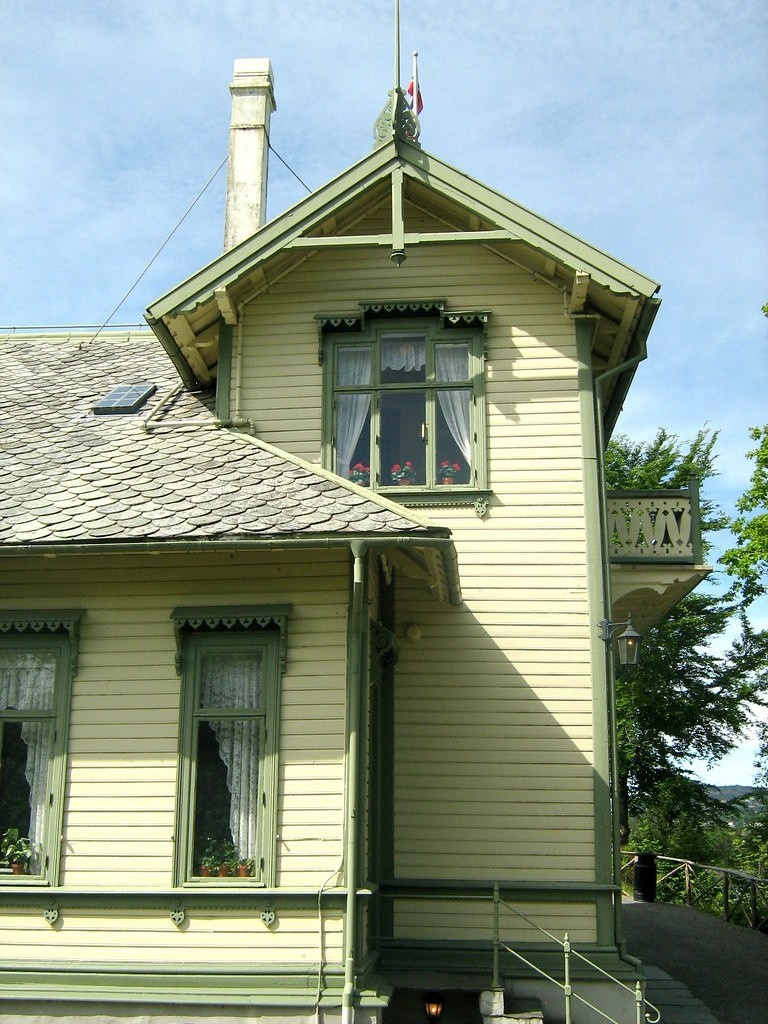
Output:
[397,477,412,486]
[357,480,367,486]
[2,828,33,875]
[199,836,251,876]
[442,477,454,485]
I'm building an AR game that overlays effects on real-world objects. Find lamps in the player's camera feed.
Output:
[608,610,642,665]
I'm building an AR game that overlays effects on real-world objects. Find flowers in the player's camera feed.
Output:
[349,464,371,483]
[391,460,417,482]
[437,460,461,479]
